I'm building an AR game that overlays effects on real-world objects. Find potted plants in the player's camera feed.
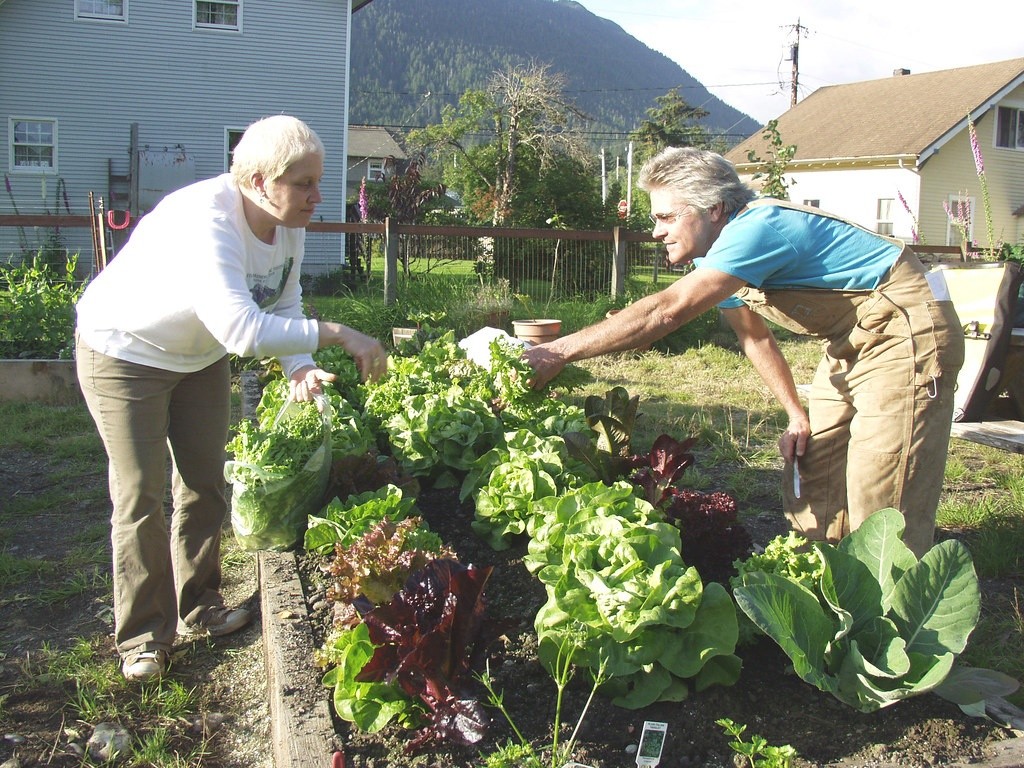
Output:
[392,308,447,347]
[511,239,563,347]
[606,297,650,356]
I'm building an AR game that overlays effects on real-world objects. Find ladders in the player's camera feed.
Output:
[108,156,132,264]
[91,229,115,281]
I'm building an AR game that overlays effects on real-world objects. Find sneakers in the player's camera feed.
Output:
[123,649,166,681]
[176,604,250,636]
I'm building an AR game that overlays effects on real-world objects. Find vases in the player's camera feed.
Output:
[719,310,733,333]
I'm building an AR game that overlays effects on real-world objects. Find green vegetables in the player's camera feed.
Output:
[224,402,328,550]
[485,333,596,411]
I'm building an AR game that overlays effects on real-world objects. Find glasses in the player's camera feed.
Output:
[648,203,689,225]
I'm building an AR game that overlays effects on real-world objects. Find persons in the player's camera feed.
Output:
[75,115,387,683]
[518,146,966,561]
